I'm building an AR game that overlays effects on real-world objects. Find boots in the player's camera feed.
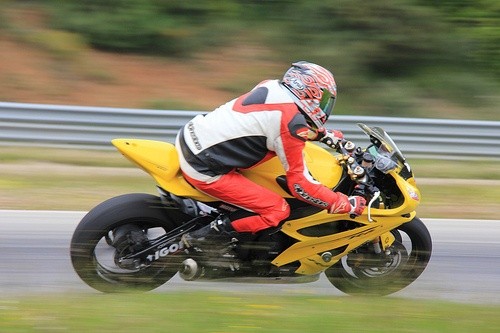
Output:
[180,213,238,259]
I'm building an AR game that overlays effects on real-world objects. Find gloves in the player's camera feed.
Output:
[348,195,366,217]
[320,129,343,148]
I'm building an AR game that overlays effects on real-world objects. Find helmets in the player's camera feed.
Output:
[282,61,337,132]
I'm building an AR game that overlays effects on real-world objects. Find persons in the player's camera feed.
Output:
[173,60,367,254]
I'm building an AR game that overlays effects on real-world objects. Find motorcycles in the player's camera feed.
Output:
[69,122,433,297]
